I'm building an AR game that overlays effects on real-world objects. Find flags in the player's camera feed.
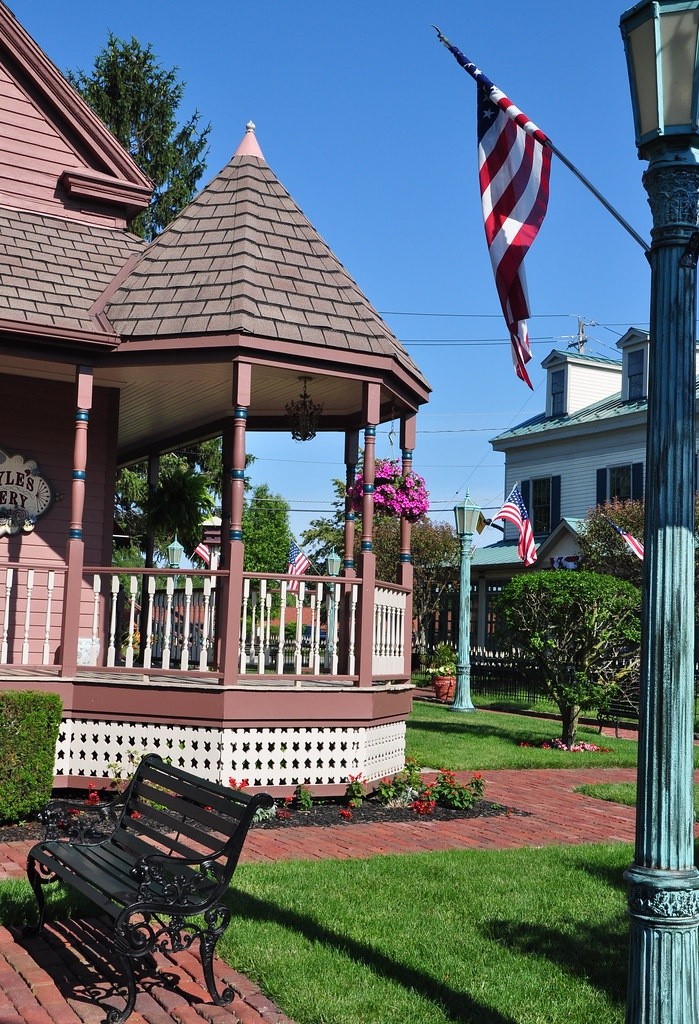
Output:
[451,46,554,392]
[601,510,644,561]
[287,540,312,594]
[491,483,538,565]
[195,542,211,565]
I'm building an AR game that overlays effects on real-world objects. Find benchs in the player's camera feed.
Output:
[597,675,641,737]
[22,754,274,1024]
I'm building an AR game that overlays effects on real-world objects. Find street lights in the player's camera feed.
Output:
[165,533,184,589]
[448,485,482,713]
[619,0,698,1024]
[325,546,342,657]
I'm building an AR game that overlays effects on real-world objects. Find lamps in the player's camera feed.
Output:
[285,376,325,441]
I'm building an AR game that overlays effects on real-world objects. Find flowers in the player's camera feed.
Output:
[426,663,456,676]
[348,457,431,524]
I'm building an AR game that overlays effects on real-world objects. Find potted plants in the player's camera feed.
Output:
[426,641,456,701]
